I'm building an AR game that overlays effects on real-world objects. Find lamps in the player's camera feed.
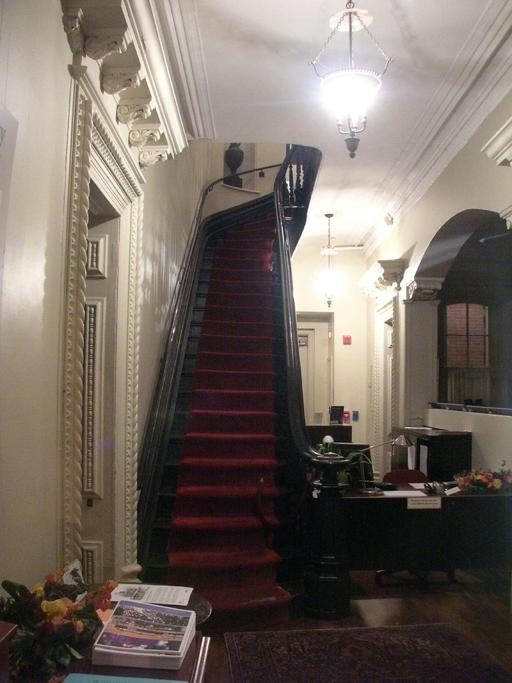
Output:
[313,5,390,160]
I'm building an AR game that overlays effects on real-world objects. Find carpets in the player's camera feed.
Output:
[223,623,511,680]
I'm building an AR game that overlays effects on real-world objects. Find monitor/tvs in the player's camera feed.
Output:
[331,443,373,490]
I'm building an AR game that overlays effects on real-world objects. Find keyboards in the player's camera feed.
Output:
[357,482,398,489]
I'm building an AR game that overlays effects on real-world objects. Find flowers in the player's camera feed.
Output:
[451,466,512,495]
[0,574,99,682]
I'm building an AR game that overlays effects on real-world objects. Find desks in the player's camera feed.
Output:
[60,581,215,681]
[315,490,512,584]
[306,424,373,484]
[389,424,472,476]
